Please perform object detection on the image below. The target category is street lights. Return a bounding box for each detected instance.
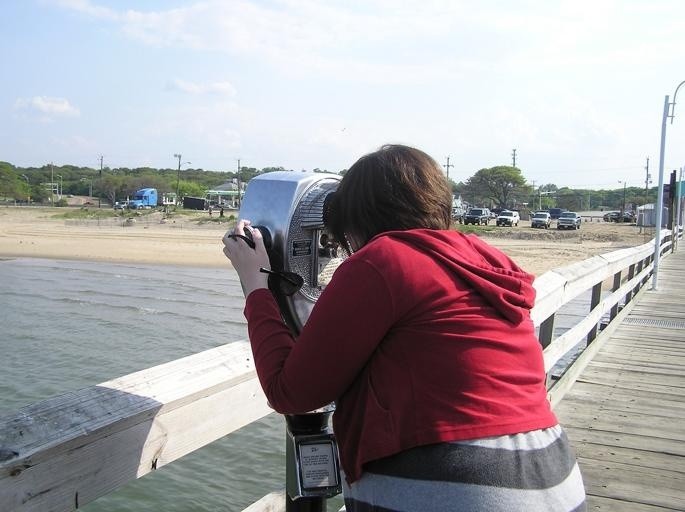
[617,176,631,217]
[93,151,109,189]
[509,146,521,175]
[171,153,193,197]
[641,153,654,210]
[442,156,456,182]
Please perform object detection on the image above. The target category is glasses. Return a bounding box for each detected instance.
[228,225,305,297]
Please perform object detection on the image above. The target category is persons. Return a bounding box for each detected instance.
[221,144,587,512]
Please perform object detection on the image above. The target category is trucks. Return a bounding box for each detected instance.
[130,187,158,210]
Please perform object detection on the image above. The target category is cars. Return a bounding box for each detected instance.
[451,207,583,231]
[113,201,129,210]
[603,211,636,224]
